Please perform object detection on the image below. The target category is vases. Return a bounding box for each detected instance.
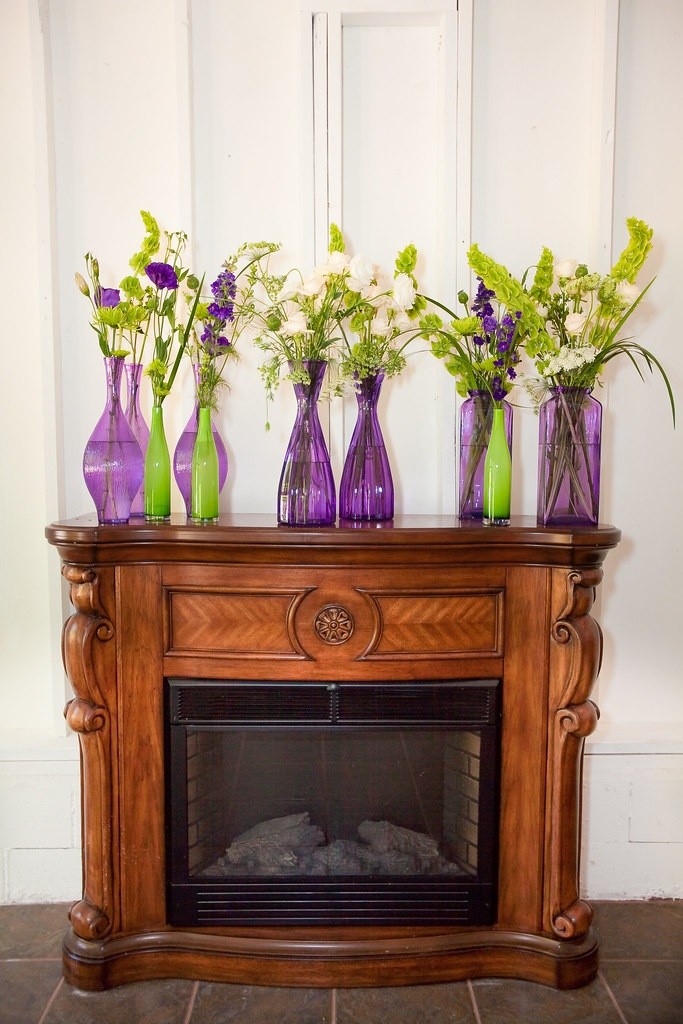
[484,405,513,520]
[455,387,513,520]
[173,401,227,516]
[119,362,148,518]
[339,365,396,523]
[532,385,602,528]
[277,358,337,527]
[146,404,170,516]
[191,406,219,522]
[81,352,143,526]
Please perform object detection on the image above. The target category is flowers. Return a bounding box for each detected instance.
[74,208,676,425]
